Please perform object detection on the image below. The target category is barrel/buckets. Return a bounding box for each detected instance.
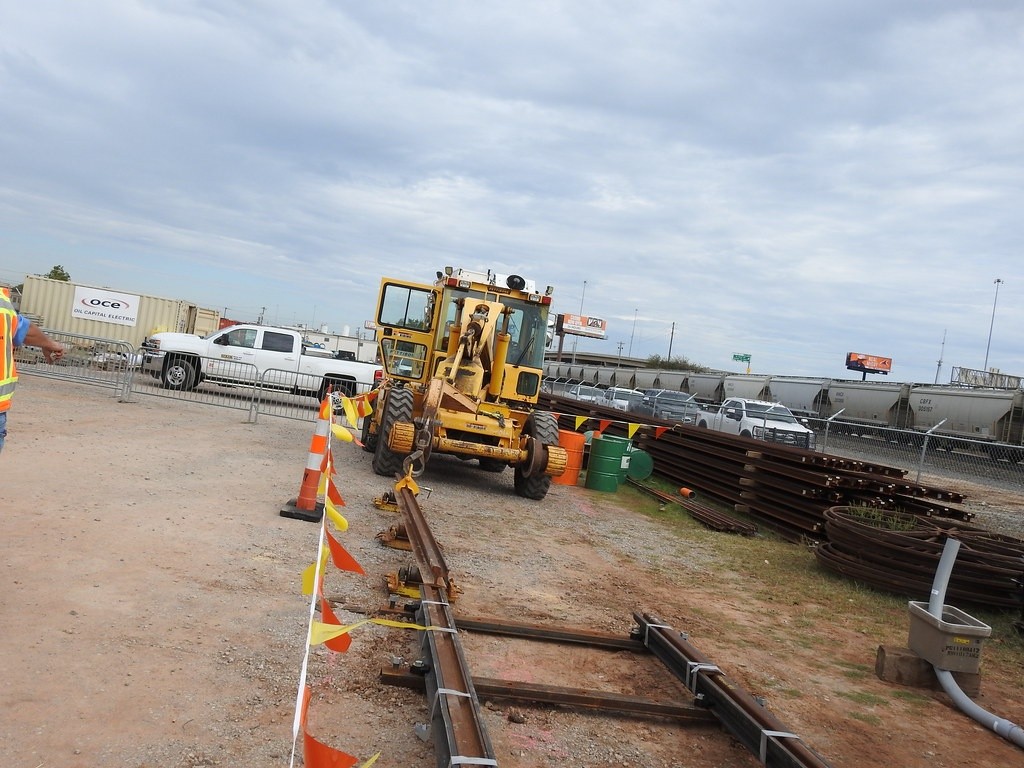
[551,429,586,486]
[603,434,633,485]
[628,447,654,481]
[585,437,625,492]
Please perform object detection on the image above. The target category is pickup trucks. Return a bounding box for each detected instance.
[696,398,816,452]
[594,387,644,417]
[142,325,382,415]
[628,390,695,425]
[562,385,604,404]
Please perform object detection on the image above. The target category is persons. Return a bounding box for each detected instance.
[0,285,63,454]
[850,355,867,368]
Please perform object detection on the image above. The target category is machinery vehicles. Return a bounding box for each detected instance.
[362,266,566,502]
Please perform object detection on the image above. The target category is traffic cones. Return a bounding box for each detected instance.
[279,382,341,523]
